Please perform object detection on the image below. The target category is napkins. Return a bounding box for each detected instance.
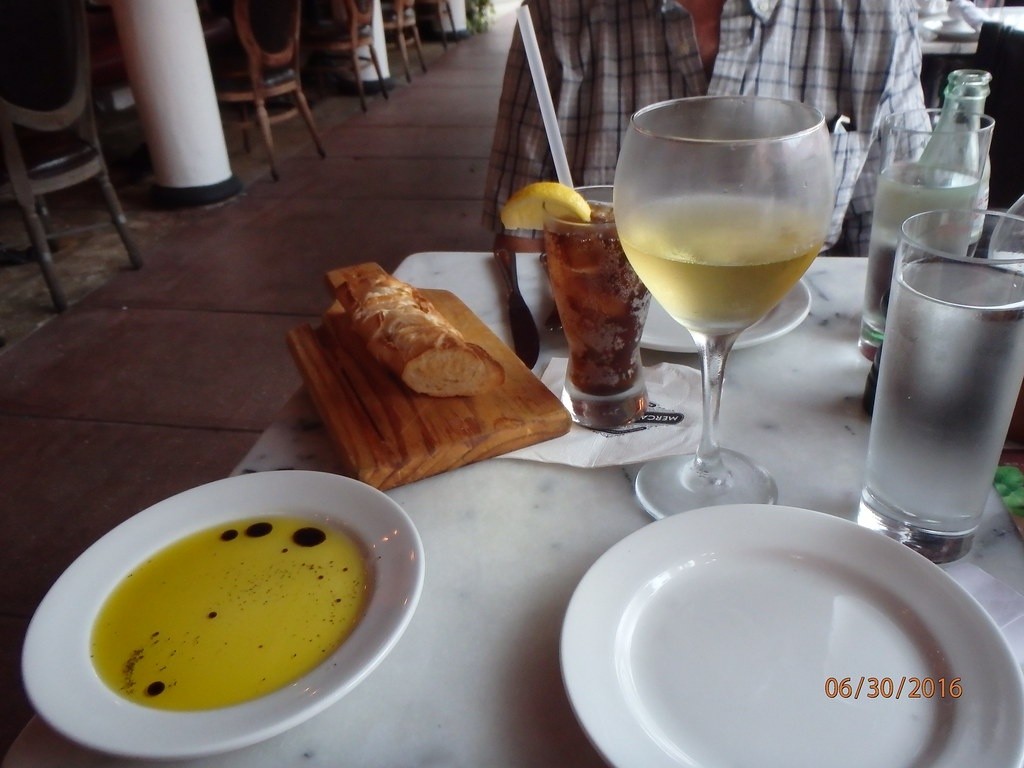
[494,357,725,469]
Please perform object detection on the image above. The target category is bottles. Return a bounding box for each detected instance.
[912,71,997,257]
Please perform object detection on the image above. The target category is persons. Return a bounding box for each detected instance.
[478,0,932,261]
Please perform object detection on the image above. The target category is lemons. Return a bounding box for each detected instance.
[502,182,591,231]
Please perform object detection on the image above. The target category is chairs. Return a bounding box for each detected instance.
[0,0,457,313]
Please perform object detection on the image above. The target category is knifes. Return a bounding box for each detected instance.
[495,250,540,371]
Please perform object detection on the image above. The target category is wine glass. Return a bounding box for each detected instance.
[611,96,835,522]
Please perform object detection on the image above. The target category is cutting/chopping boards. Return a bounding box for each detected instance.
[288,262,571,494]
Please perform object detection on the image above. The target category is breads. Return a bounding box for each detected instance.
[336,273,504,396]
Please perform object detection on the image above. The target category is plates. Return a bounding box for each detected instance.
[21,470,426,761]
[635,277,814,354]
[558,504,1024,768]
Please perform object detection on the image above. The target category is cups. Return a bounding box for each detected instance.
[858,108,995,357]
[856,207,1024,563]
[542,184,648,429]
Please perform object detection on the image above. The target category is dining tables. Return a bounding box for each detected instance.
[0,252,1024,768]
